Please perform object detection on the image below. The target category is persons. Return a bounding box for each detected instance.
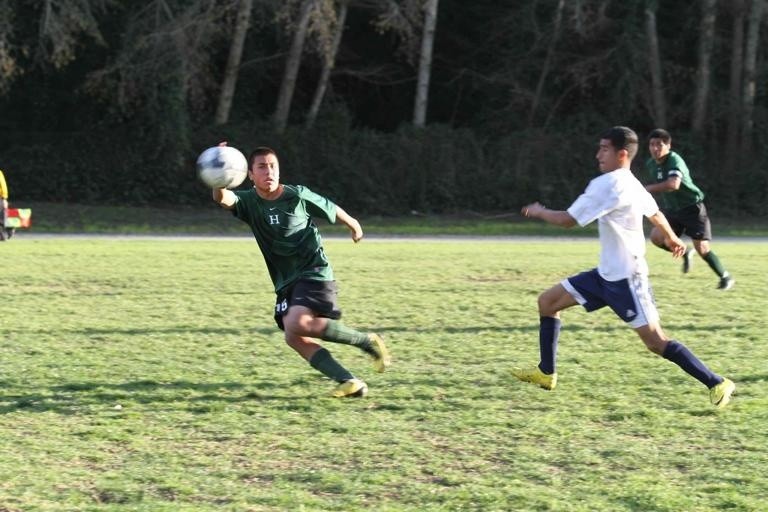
[0,170,15,240]
[509,126,736,412]
[212,141,388,398]
[644,128,735,291]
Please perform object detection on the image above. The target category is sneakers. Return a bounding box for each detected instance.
[716,275,735,289]
[709,377,735,408]
[510,366,557,390]
[683,248,696,273]
[327,378,368,398]
[365,333,388,373]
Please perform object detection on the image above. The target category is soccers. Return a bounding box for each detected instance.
[199,145,248,188]
